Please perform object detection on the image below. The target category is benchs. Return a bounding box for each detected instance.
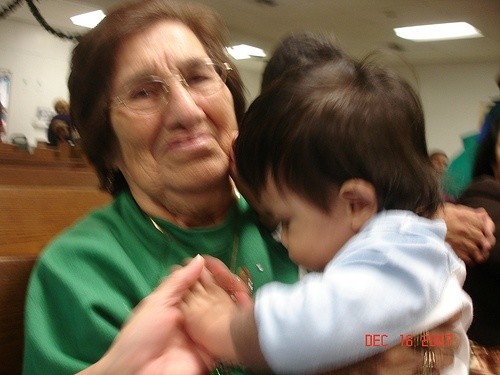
[0,141,116,375]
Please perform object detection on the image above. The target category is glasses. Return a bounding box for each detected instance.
[107,62,231,115]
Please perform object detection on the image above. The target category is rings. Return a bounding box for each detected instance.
[422,350,435,370]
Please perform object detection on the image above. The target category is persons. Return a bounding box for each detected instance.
[19,0,496,375]
[70,253,251,375]
[427,86,499,352]
[166,43,478,375]
[48,100,82,147]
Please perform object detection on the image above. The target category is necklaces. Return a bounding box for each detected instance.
[146,193,242,274]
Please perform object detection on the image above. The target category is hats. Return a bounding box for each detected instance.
[427,141,448,158]
[53,120,67,130]
